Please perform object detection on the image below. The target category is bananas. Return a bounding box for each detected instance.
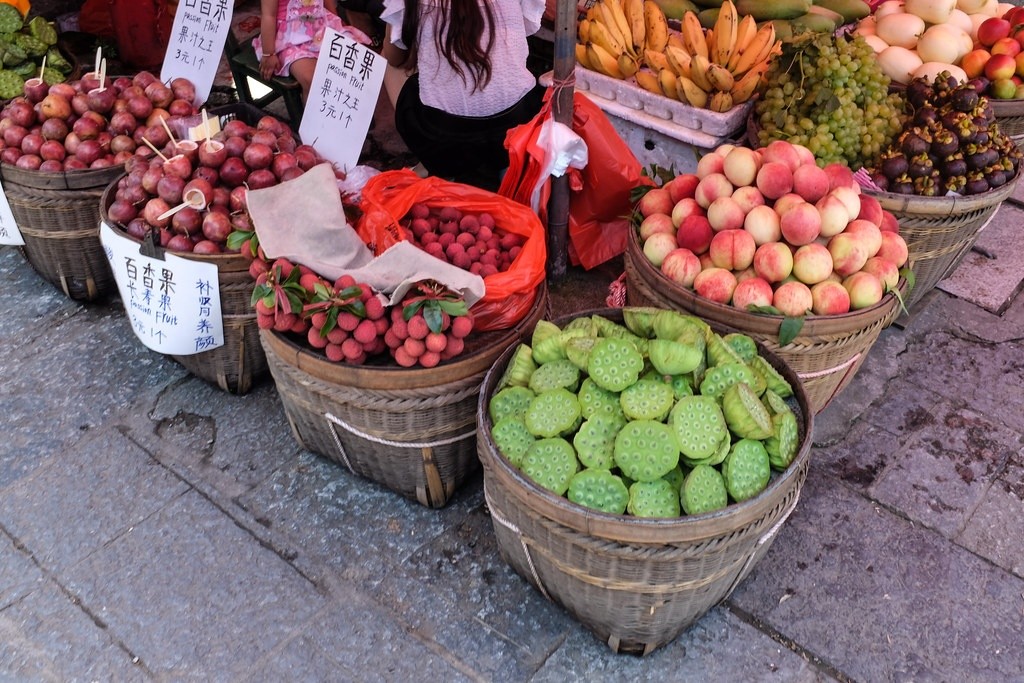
[574,0,785,108]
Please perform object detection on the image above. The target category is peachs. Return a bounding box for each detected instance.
[640,140,907,315]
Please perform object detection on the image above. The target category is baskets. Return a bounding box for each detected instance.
[98,130,339,396]
[834,0,1024,151]
[253,206,551,510]
[14,26,127,84]
[624,178,908,418]
[0,75,163,300]
[748,89,1024,313]
[476,305,816,657]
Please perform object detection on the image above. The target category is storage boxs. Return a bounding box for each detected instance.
[539,69,746,185]
[574,59,753,136]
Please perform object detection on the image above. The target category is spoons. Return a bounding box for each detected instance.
[201,107,216,154]
[156,192,203,222]
[158,114,184,149]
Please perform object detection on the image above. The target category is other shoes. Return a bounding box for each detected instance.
[402,166,456,182]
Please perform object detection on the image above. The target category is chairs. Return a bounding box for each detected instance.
[221,0,303,131]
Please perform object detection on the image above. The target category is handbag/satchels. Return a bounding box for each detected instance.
[358,166,546,329]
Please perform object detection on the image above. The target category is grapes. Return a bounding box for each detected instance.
[752,30,913,169]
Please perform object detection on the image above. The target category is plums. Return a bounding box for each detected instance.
[961,6,1023,100]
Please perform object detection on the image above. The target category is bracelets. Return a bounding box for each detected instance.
[261,53,275,56]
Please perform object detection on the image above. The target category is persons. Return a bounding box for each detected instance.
[379,0,546,179]
[252,0,374,155]
